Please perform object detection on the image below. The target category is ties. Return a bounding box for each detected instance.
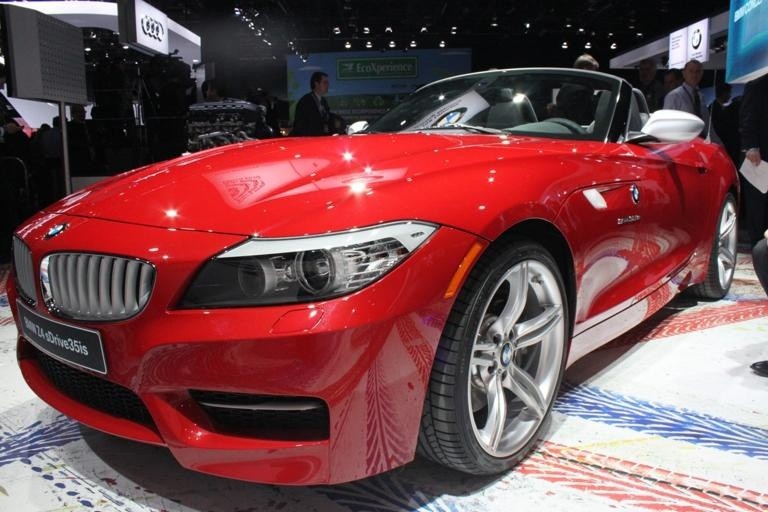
[691,89,701,117]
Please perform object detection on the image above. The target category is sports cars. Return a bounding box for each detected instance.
[5,68,745,489]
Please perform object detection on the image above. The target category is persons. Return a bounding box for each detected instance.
[0,62,97,263]
[206,78,232,99]
[292,71,333,136]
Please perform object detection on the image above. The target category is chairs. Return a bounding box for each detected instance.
[486,89,538,133]
[591,86,650,142]
